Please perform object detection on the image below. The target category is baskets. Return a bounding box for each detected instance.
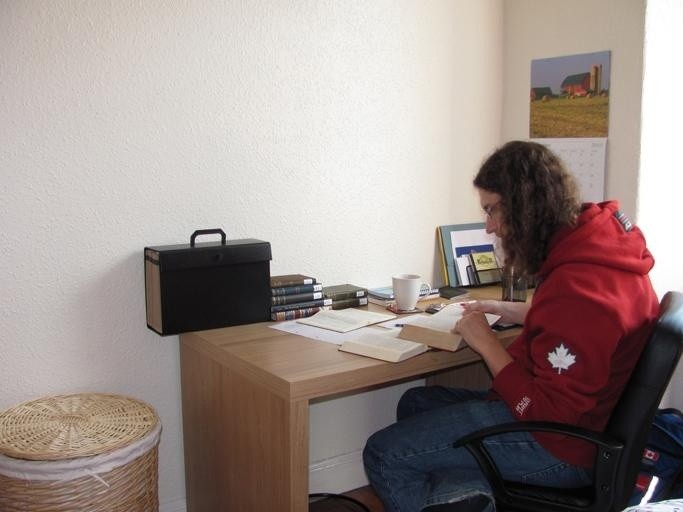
[0,393,162,512]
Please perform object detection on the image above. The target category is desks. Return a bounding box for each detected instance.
[177,288,548,512]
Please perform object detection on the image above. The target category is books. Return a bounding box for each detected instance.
[336,320,432,361]
[439,251,502,299]
[399,303,503,354]
[271,274,370,321]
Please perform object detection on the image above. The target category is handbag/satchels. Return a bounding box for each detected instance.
[627,407,683,505]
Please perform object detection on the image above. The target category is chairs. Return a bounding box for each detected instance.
[452,291,682,511]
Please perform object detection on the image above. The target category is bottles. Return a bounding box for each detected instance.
[500,256,528,303]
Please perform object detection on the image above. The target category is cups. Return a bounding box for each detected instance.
[391,274,432,312]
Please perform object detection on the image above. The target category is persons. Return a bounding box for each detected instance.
[363,141,660,511]
